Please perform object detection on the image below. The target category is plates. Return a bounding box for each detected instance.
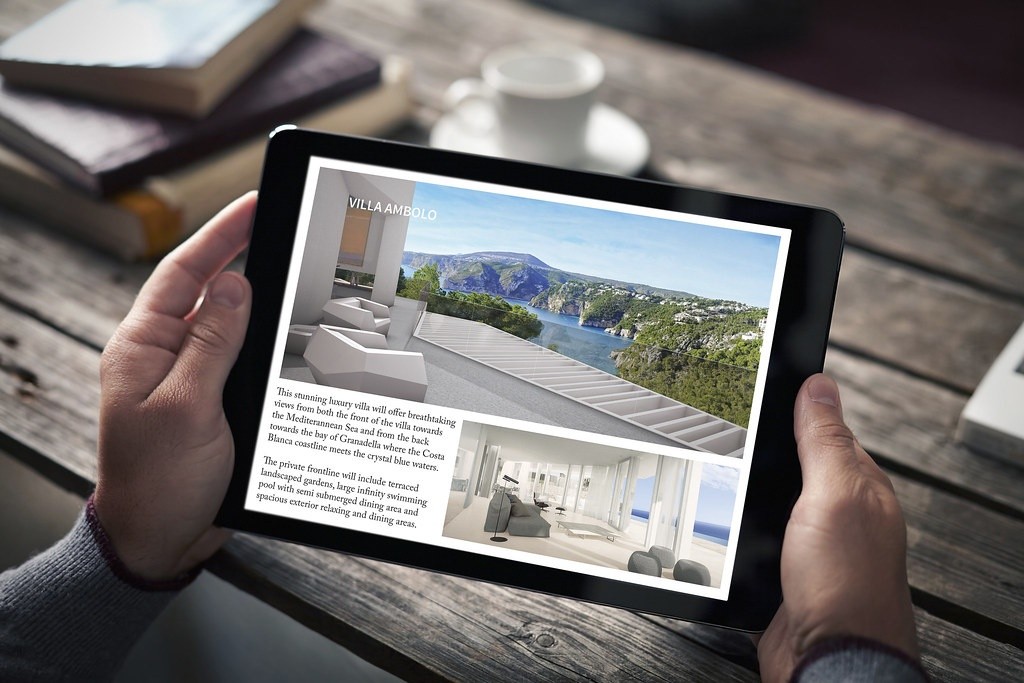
[425,96,656,182]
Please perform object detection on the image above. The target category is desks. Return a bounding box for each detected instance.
[2,1,1024,683]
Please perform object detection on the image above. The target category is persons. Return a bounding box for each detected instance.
[0,192,934,682]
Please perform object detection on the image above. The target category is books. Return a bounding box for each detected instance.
[1,2,411,263]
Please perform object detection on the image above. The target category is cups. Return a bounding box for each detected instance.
[445,39,606,169]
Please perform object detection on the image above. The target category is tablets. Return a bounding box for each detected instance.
[210,125,847,631]
